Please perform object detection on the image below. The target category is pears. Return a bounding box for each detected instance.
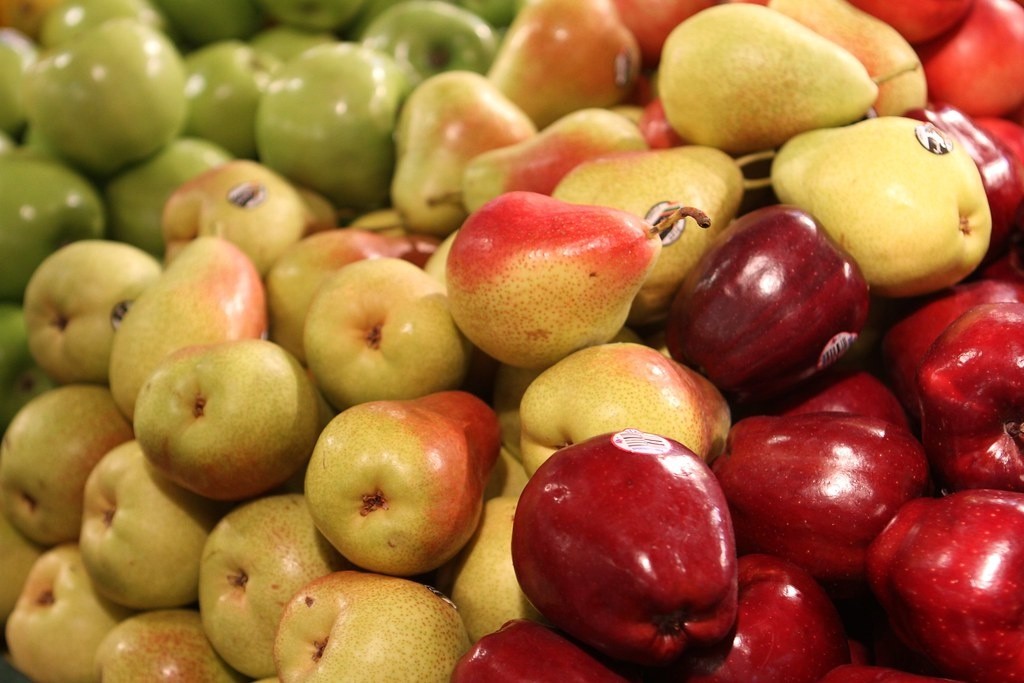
[0,0,992,683]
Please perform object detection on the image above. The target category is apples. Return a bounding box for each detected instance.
[451,1,1024,683]
